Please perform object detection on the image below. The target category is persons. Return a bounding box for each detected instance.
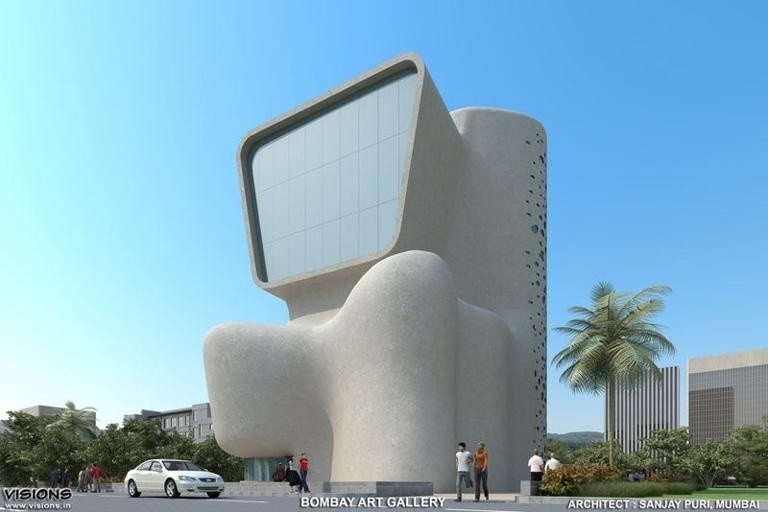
[296,451,312,493]
[288,454,300,494]
[544,452,561,473]
[527,448,544,480]
[472,439,490,503]
[452,441,474,502]
[48,462,101,492]
